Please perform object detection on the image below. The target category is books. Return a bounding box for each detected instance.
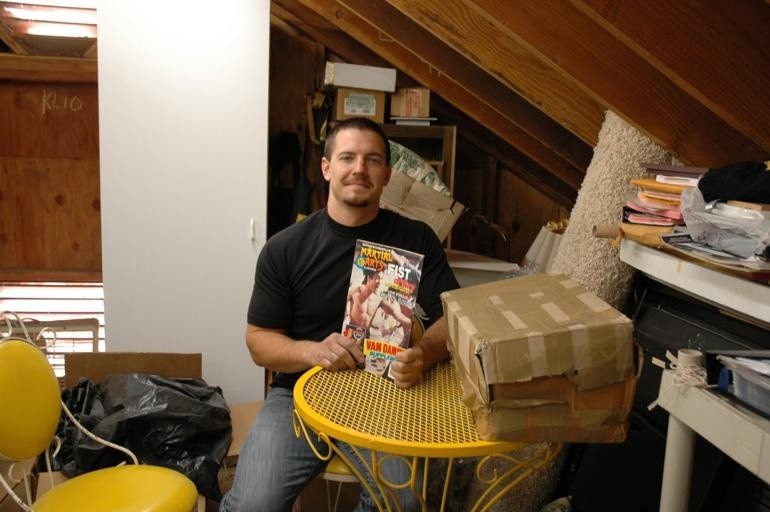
[322,57,432,126]
[436,272,636,445]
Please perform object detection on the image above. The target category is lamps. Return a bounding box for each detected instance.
[0,313,202,511]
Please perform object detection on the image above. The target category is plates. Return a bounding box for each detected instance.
[620,238,770,511]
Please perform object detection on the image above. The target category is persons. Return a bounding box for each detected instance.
[219,116,460,512]
[346,270,412,352]
[381,287,403,328]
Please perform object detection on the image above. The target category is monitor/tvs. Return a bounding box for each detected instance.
[340,238,426,383]
